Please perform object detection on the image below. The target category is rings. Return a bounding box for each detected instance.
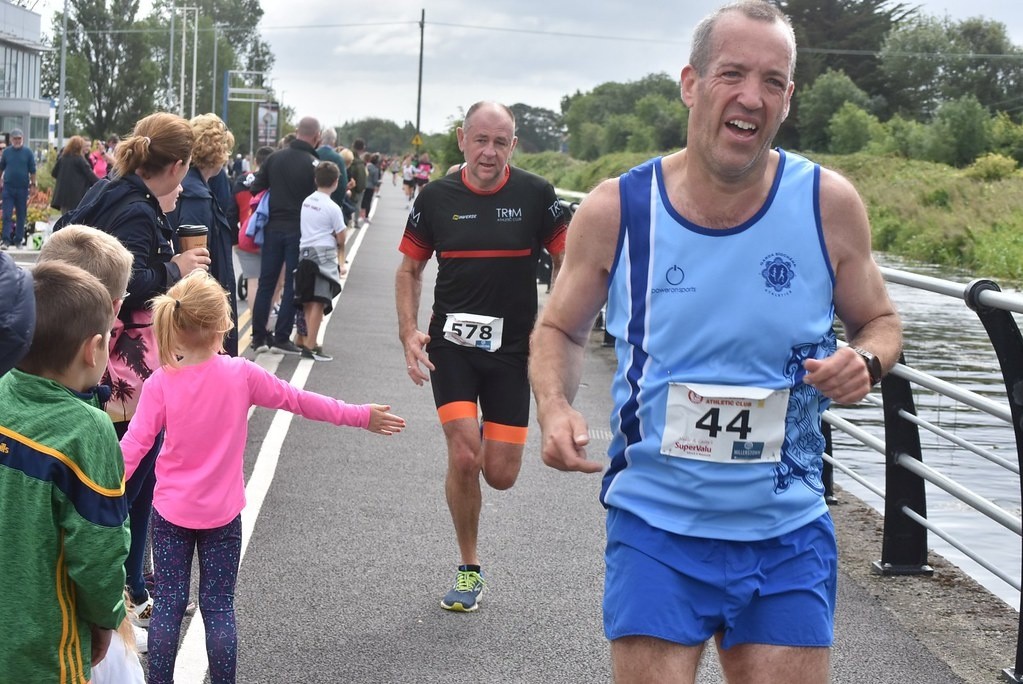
[408,365,412,369]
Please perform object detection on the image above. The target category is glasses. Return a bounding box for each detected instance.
[13,137,22,140]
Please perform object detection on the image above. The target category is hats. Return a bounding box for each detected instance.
[10,128,23,138]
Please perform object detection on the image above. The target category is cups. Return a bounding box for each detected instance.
[175,224,209,254]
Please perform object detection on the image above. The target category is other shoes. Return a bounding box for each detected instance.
[405,205,410,210]
[1,241,10,250]
[13,236,22,250]
[364,217,373,224]
[123,585,154,628]
[354,224,361,229]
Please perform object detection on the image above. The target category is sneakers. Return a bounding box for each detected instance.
[294,345,304,351]
[254,340,269,353]
[304,349,333,361]
[440,571,490,612]
[271,344,304,355]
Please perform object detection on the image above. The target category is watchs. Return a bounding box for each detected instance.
[854,347,882,386]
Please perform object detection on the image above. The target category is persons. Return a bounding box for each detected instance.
[394,100,570,610]
[119,269,406,684]
[233,117,388,361]
[0,112,243,684]
[390,151,434,210]
[529,0,903,684]
